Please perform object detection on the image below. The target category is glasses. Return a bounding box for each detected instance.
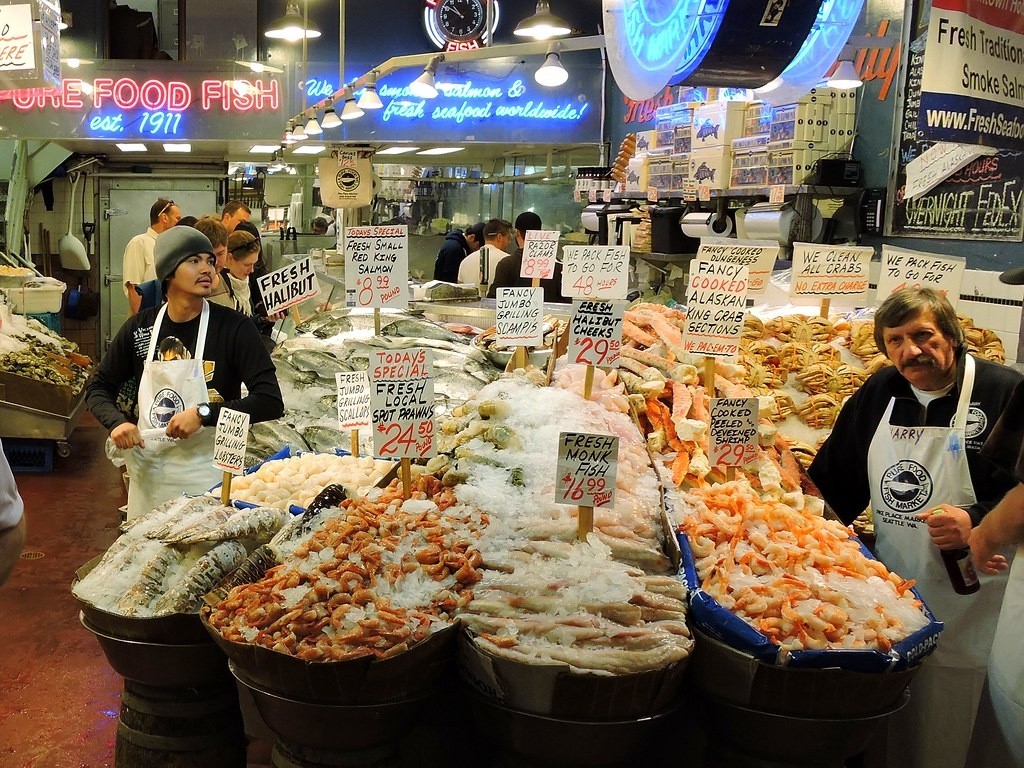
[157,199,174,219]
[230,236,261,256]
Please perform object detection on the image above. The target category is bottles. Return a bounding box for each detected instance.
[665,298,687,313]
[574,167,618,192]
[932,509,981,595]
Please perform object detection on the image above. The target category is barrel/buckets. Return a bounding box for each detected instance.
[268,733,409,768]
[114,679,249,768]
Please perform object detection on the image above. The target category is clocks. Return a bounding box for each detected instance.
[423,0,500,52]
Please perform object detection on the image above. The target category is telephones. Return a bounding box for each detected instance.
[856,184,883,233]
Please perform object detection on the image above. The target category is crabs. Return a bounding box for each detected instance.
[633,304,1008,486]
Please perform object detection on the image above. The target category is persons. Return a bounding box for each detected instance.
[0,438,26,587]
[486,212,572,304]
[808,288,1024,768]
[175,200,289,356]
[312,207,336,235]
[457,218,513,298]
[433,222,486,284]
[85,226,285,522]
[123,199,182,321]
[967,377,1024,768]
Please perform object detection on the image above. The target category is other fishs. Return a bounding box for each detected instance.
[219,295,569,490]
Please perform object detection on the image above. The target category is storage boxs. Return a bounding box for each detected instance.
[0,276,68,335]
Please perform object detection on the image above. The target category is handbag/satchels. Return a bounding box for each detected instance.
[64,274,98,321]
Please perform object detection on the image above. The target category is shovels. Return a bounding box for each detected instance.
[58,171,91,271]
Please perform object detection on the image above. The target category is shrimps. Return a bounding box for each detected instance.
[82,488,923,671]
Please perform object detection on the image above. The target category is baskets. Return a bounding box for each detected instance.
[25,312,61,337]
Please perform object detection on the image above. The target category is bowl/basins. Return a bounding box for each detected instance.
[683,685,911,760]
[463,688,688,768]
[225,659,456,752]
[78,609,228,687]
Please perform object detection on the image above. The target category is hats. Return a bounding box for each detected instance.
[154,223,216,287]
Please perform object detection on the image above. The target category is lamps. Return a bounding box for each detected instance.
[60,55,96,68]
[513,0,571,38]
[281,118,298,144]
[303,105,323,134]
[827,36,900,88]
[408,54,445,98]
[289,112,309,140]
[321,95,343,129]
[267,144,287,171]
[1,437,55,473]
[340,83,365,120]
[626,85,856,193]
[356,70,383,110]
[534,41,569,87]
[264,0,322,42]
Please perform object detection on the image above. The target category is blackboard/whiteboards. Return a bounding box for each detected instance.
[884,2,1021,243]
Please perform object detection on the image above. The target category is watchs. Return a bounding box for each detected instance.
[197,402,212,427]
